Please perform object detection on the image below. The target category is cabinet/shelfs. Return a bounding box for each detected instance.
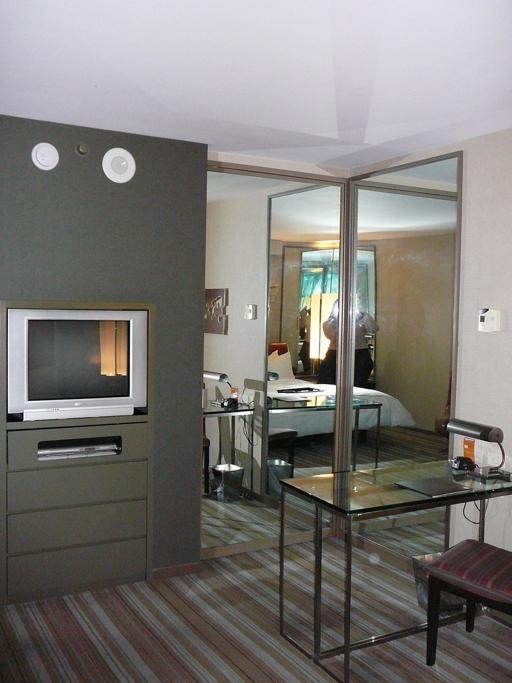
[0,298,156,605]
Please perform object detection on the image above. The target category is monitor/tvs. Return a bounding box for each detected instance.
[8,309,147,422]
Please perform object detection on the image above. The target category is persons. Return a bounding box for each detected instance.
[318,292,374,389]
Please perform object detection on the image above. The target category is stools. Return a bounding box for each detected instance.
[267,424,298,479]
[424,538,512,667]
[202,436,211,494]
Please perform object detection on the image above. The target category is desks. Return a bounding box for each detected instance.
[201,397,256,501]
[277,456,512,683]
[268,394,384,472]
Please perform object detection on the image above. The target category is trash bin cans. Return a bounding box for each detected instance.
[266,459,292,495]
[213,463,244,501]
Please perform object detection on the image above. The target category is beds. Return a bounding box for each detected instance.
[267,343,416,452]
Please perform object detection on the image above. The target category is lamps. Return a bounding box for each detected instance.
[443,419,506,480]
[201,369,239,409]
[266,369,280,382]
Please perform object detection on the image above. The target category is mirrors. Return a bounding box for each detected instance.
[260,183,344,529]
[200,158,349,562]
[347,150,464,537]
[344,177,457,533]
